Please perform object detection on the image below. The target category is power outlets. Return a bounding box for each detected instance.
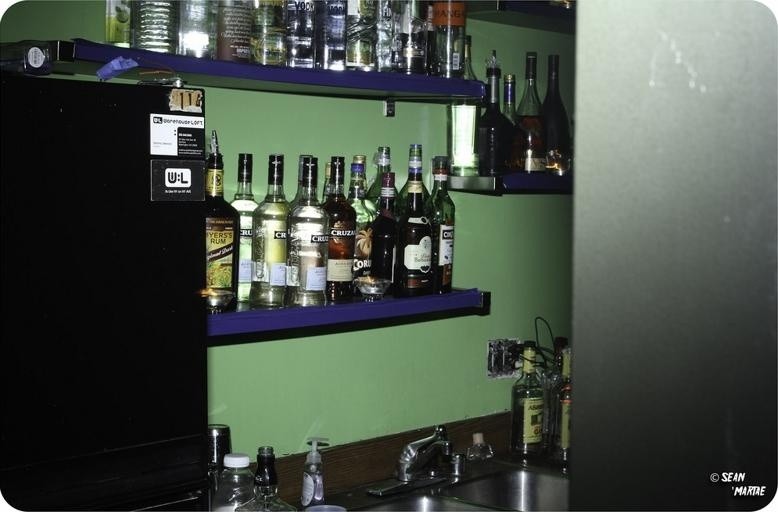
[485,338,522,381]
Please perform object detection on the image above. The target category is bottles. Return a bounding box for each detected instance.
[204,143,458,311]
[447,34,570,177]
[232,445,299,512]
[466,433,494,459]
[511,333,571,471]
[131,0,471,80]
[212,451,255,512]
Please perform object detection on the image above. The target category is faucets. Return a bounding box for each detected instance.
[391,431,452,483]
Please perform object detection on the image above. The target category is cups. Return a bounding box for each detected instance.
[306,504,346,511]
[207,422,232,490]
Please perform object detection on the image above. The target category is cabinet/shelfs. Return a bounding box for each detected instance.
[445,1,577,197]
[0,34,486,338]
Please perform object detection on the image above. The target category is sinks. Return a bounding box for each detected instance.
[442,469,569,512]
[366,494,493,512]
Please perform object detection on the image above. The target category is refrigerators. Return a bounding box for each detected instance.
[1,75,204,508]
